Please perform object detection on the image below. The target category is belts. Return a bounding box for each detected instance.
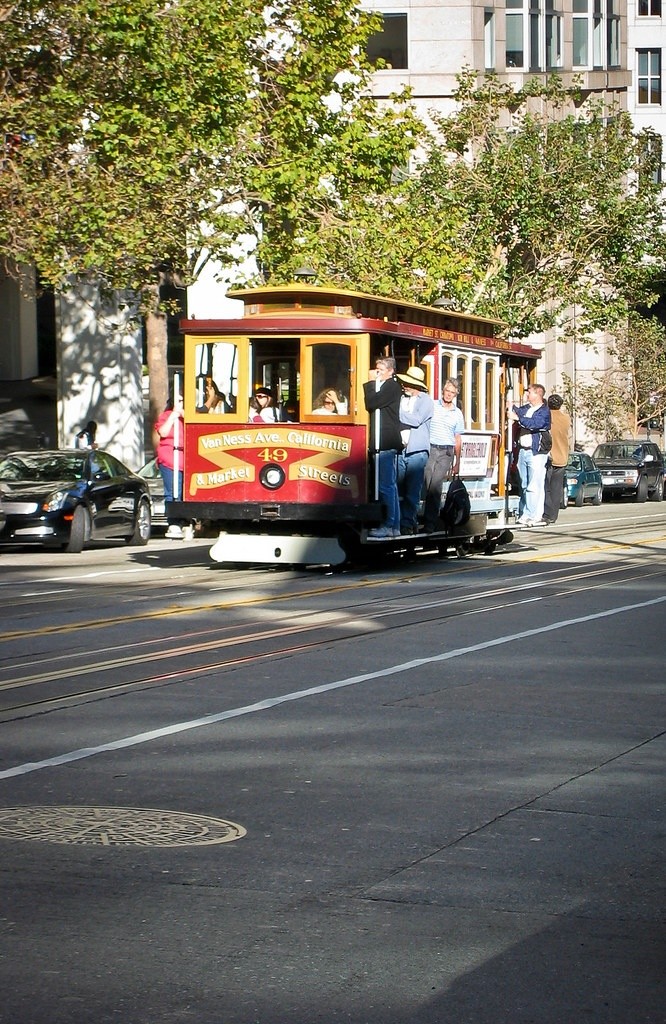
[521,446,532,450]
[431,445,454,449]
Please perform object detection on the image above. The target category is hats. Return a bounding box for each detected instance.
[396,366,428,388]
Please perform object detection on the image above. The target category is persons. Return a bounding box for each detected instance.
[363,356,404,536]
[155,390,193,542]
[506,383,551,525]
[249,388,279,423]
[396,367,434,535]
[312,388,348,415]
[542,394,571,523]
[418,378,465,534]
[197,375,230,413]
[75,420,98,467]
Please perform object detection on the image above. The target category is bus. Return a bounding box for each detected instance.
[165,285,542,574]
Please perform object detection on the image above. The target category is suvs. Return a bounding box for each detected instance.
[592,440,664,503]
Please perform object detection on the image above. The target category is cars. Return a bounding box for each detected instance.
[137,456,207,538]
[564,452,603,506]
[0,449,152,553]
[490,450,568,510]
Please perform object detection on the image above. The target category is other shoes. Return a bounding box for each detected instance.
[418,528,435,534]
[516,514,531,523]
[165,525,190,538]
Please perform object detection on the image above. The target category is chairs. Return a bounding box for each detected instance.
[633,447,643,458]
[572,461,580,468]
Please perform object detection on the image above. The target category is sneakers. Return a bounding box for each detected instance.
[401,528,413,534]
[370,527,393,536]
[393,529,401,536]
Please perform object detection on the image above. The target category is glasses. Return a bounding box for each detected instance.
[256,395,268,398]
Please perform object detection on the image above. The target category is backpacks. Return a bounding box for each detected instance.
[444,473,470,527]
